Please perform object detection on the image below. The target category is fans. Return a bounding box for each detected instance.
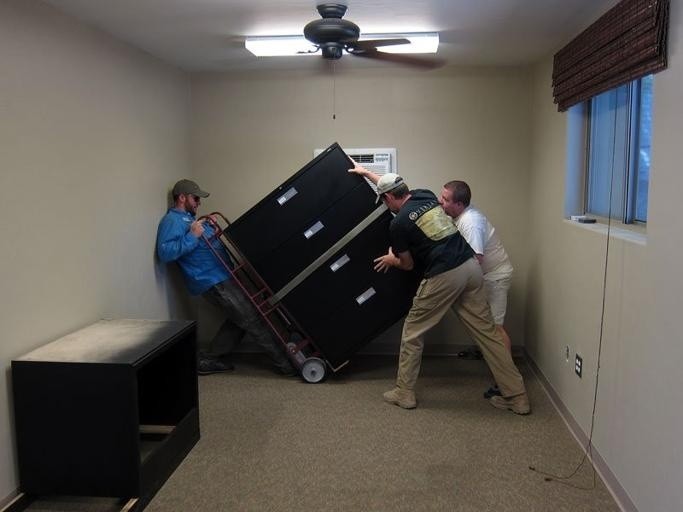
[296,3,447,70]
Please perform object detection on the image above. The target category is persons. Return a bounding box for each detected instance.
[347,154,530,414]
[437,180,514,399]
[155,178,302,378]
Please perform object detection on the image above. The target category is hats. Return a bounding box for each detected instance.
[172,180,210,199]
[374,173,404,205]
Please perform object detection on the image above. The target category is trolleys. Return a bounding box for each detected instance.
[194,211,351,386]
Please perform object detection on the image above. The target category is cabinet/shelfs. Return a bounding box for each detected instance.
[10,316,200,498]
[220,141,424,381]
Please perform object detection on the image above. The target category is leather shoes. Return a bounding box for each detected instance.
[457,350,482,360]
[484,388,501,397]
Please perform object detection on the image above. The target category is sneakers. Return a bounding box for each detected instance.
[490,393,531,415]
[280,367,298,377]
[198,351,234,375]
[383,387,417,409]
